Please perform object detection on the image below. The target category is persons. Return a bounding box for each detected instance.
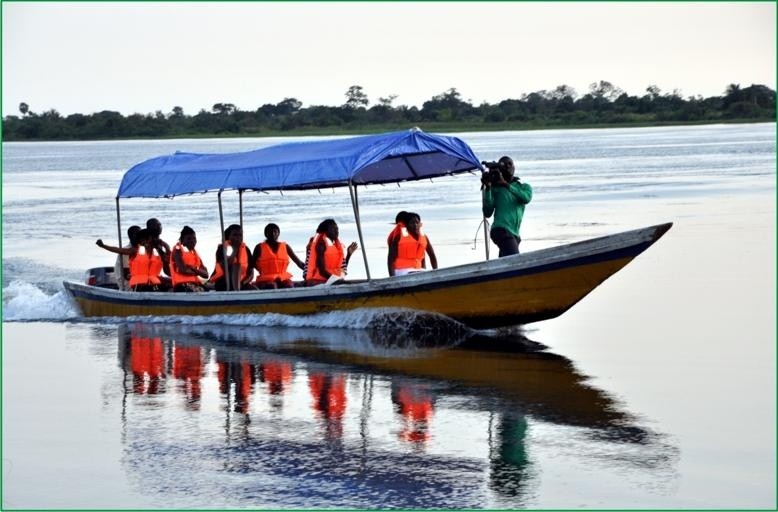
[257,357,295,409]
[388,211,426,270]
[253,223,305,289]
[390,377,436,443]
[387,212,437,277]
[303,219,358,287]
[307,366,348,439]
[118,322,256,425]
[170,226,209,293]
[488,408,531,498]
[96,218,170,292]
[208,224,254,291]
[481,156,532,258]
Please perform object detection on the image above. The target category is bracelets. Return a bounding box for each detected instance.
[196,266,200,272]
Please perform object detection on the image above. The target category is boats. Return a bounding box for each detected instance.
[129,323,653,445]
[58,222,676,330]
